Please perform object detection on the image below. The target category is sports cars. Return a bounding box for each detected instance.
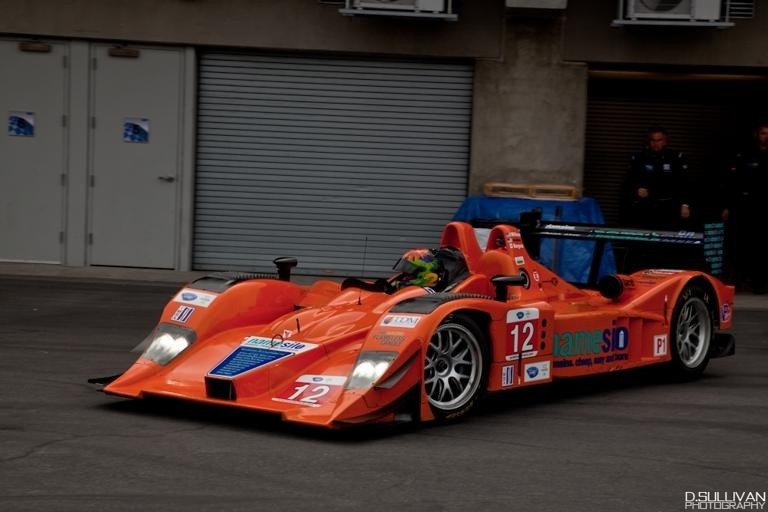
[85,206,739,436]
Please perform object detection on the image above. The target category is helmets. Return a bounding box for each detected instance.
[392,248,442,290]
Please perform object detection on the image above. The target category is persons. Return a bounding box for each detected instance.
[624,123,768,294]
